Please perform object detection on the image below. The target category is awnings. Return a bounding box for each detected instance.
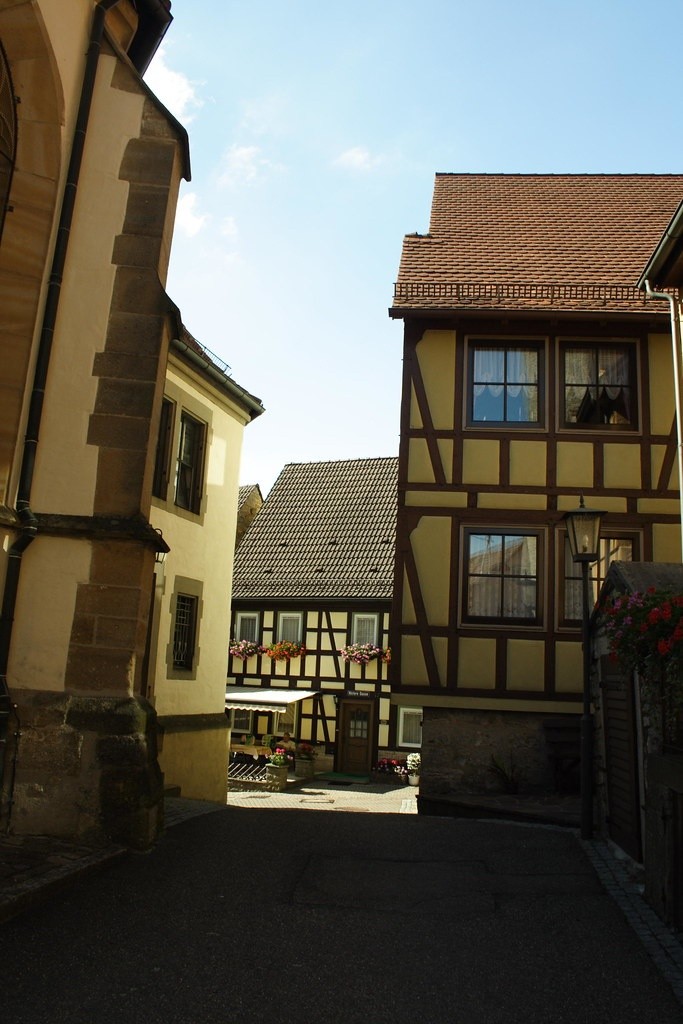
[225,685,321,713]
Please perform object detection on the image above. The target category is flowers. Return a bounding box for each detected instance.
[594,585,683,676]
[381,646,393,664]
[269,748,294,769]
[229,638,265,661]
[341,642,380,664]
[378,753,421,774]
[266,639,305,662]
[298,743,316,760]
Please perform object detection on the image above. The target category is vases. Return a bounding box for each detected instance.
[408,774,419,785]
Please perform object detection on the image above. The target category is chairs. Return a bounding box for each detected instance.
[241,735,255,744]
[261,734,274,746]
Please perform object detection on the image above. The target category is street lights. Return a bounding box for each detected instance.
[559,494,609,840]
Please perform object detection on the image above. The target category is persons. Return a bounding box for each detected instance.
[276,732,296,753]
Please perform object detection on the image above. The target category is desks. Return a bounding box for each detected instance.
[230,744,273,766]
